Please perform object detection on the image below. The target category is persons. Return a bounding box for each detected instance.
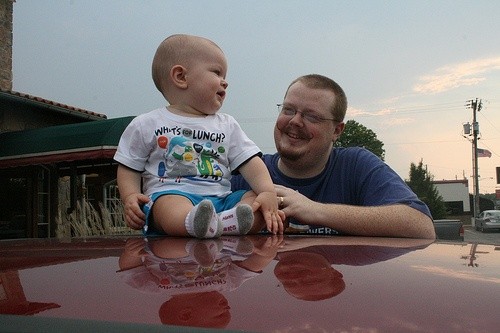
[116,236,286,328]
[272,237,438,301]
[230,74,436,239]
[113,34,285,238]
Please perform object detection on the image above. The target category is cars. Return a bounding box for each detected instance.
[475,210,500,233]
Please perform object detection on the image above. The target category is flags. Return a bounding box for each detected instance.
[477,148,492,158]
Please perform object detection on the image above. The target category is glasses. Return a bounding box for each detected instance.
[277,102,341,125]
